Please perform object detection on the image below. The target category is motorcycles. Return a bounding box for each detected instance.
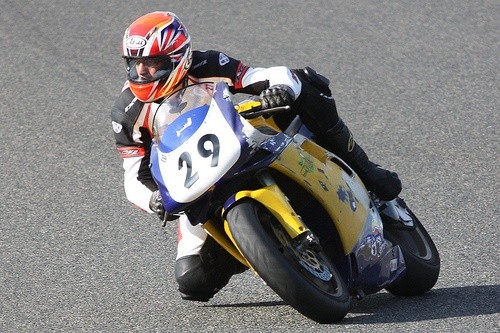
[134,81,442,324]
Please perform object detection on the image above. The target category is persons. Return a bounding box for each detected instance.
[110,11,403,301]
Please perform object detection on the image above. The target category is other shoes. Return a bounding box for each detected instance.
[361,160,401,199]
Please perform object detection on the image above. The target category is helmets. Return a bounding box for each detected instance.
[123,10,193,103]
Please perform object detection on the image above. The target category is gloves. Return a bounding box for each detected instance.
[259,84,296,119]
[149,188,181,221]
